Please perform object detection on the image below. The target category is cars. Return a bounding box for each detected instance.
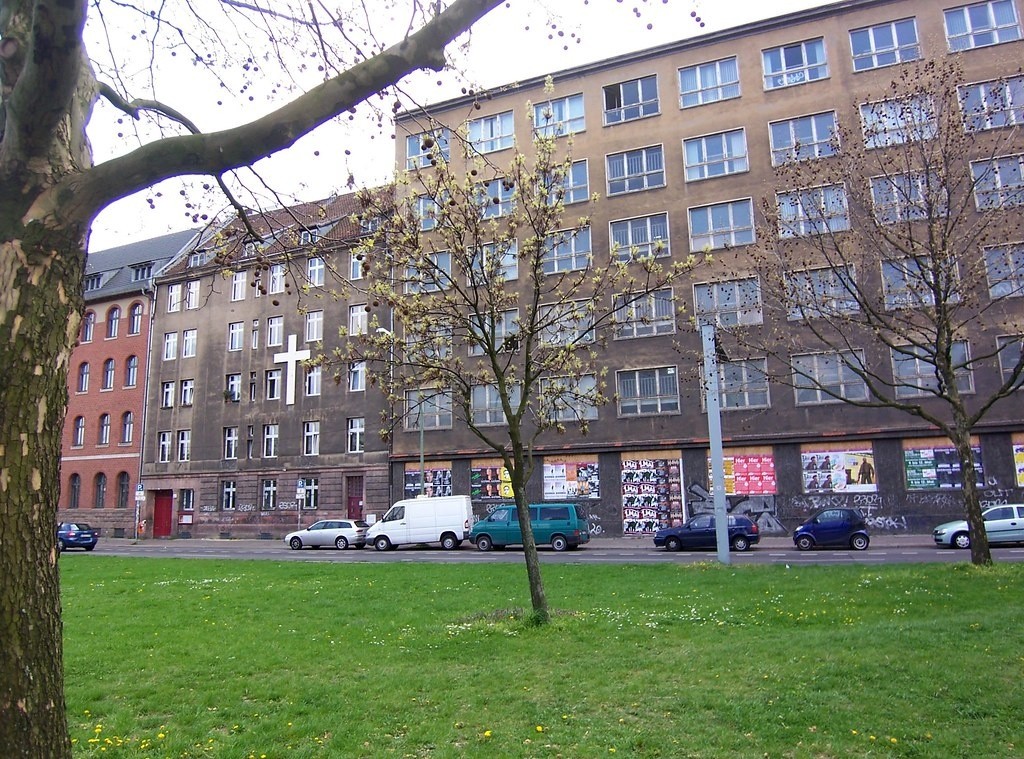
[793,507,871,552]
[932,504,1023,549]
[285,519,371,550]
[653,513,761,552]
[57,521,99,552]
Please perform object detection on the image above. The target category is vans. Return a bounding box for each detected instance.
[468,504,591,551]
[365,495,473,551]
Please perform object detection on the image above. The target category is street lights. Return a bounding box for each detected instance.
[397,397,426,495]
